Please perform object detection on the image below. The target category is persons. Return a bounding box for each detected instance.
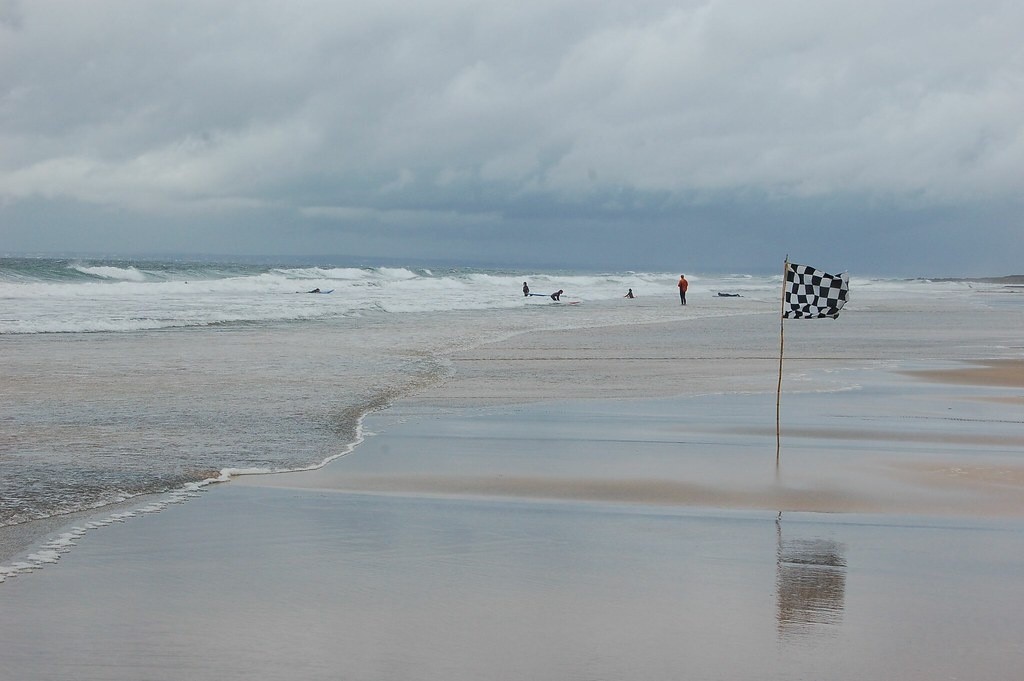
[718,291,742,299]
[523,282,530,297]
[624,288,635,299]
[677,274,689,306]
[551,290,563,302]
[296,288,320,294]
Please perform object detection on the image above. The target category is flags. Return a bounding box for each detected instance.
[782,262,849,321]
